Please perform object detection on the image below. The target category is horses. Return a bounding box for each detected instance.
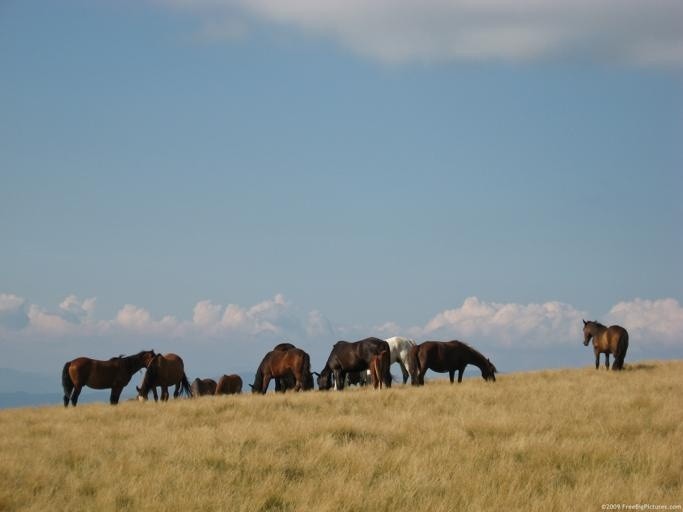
[62,348,157,408]
[583,319,629,371]
[314,336,419,390]
[189,377,217,397]
[411,340,497,387]
[214,374,243,395]
[248,343,315,395]
[135,353,194,402]
